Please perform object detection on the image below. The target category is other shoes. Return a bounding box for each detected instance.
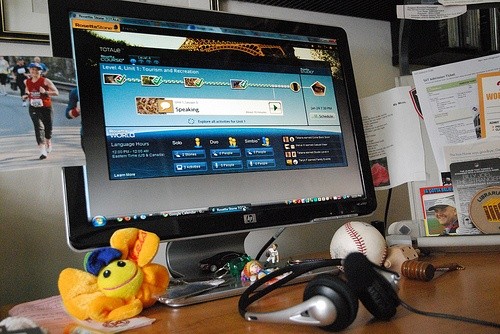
[39,150,47,159]
[23,102,27,107]
[46,145,51,152]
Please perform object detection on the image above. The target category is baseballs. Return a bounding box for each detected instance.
[330,222,385,274]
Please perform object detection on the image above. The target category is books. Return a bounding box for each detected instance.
[436,6,499,53]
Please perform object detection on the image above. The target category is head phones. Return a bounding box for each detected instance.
[238,251,401,334]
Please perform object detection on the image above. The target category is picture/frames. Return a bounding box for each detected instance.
[0,0,220,46]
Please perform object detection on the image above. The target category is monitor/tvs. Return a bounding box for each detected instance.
[47,0,378,308]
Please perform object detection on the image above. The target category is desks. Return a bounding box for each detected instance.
[0,248,499,334]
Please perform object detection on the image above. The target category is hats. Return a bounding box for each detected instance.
[428,198,455,211]
[18,57,25,60]
[30,63,43,70]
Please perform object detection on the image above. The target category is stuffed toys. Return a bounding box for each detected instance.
[239,259,280,282]
[57,228,170,323]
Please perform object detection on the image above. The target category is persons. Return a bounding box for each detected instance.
[1,56,10,96]
[32,56,50,77]
[264,243,279,266]
[65,87,85,154]
[22,63,60,158]
[13,58,29,107]
[428,198,458,233]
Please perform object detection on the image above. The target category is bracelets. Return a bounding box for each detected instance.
[72,108,80,118]
